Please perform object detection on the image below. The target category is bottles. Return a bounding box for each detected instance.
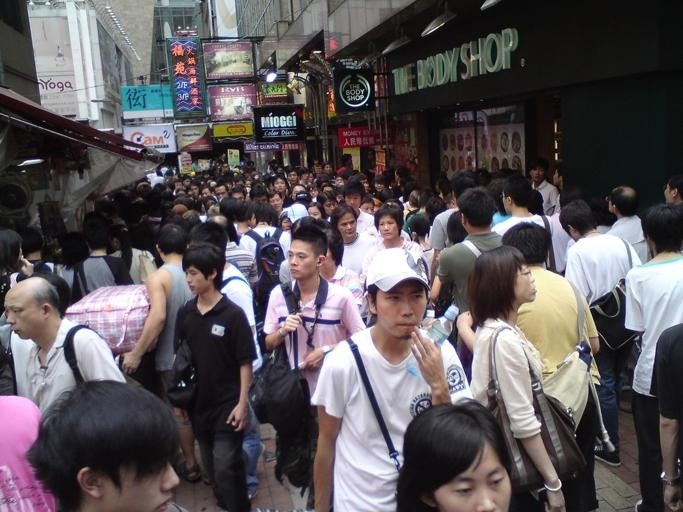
[405,303,460,380]
[421,308,436,326]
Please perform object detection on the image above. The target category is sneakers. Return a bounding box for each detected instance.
[595,444,622,466]
[635,500,644,512]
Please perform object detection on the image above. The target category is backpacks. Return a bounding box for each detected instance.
[245,227,285,316]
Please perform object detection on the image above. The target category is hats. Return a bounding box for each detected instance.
[360,248,431,300]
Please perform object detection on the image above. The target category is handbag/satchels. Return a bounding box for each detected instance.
[274,430,318,497]
[588,286,639,351]
[542,341,592,430]
[166,340,197,410]
[64,285,160,355]
[488,379,588,493]
[249,360,310,434]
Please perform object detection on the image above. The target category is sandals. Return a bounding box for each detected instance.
[175,452,201,483]
